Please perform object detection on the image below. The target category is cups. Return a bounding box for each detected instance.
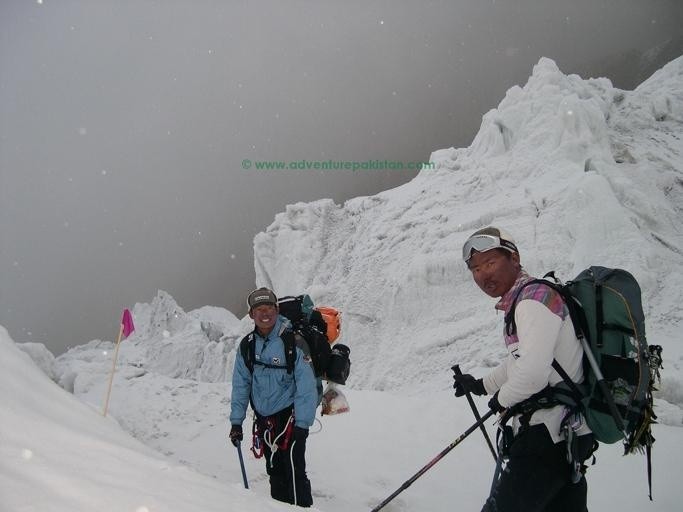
[463,235,500,263]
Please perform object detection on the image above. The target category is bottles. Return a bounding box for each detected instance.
[462,227,518,263]
[247,286,279,312]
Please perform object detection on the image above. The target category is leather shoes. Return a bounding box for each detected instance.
[291,426,311,443]
[488,388,509,416]
[452,372,489,398]
[230,422,244,447]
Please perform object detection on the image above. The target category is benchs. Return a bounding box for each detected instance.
[121,307,135,338]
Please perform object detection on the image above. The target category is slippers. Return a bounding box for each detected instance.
[240,295,332,408]
[505,265,655,444]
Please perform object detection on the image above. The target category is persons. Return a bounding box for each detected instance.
[227,286,314,509]
[450,225,597,511]
